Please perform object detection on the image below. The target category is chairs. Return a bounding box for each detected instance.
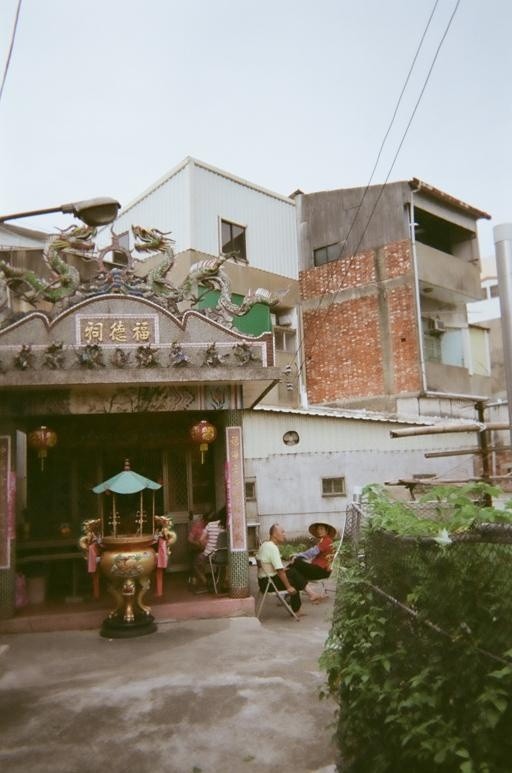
[201,532,229,597]
[252,553,299,623]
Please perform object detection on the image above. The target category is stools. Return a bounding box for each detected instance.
[301,578,329,604]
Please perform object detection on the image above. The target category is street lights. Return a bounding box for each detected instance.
[1,197,122,226]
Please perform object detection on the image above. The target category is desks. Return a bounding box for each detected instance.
[16,536,80,602]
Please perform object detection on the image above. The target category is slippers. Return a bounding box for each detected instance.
[312,595,329,605]
[293,609,308,616]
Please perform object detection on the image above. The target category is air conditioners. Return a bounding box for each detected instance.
[427,317,444,333]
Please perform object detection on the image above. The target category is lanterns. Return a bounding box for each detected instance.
[27,425,58,472]
[190,420,218,465]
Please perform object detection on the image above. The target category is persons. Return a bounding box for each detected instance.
[189,504,225,595]
[187,511,216,563]
[256,524,329,617]
[287,523,337,580]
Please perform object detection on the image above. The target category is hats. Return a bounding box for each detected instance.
[309,521,338,539]
